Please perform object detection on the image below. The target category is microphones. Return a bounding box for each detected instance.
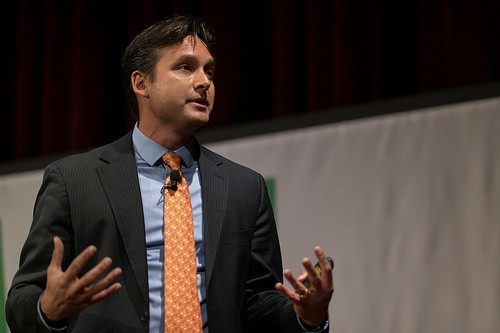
[170,169,180,189]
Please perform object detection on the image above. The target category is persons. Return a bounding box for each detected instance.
[5,12,334,333]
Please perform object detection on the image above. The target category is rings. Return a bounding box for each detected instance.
[300,291,310,299]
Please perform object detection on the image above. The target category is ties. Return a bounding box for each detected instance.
[163,150,203,333]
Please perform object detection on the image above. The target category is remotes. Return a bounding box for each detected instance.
[302,256,333,289]
